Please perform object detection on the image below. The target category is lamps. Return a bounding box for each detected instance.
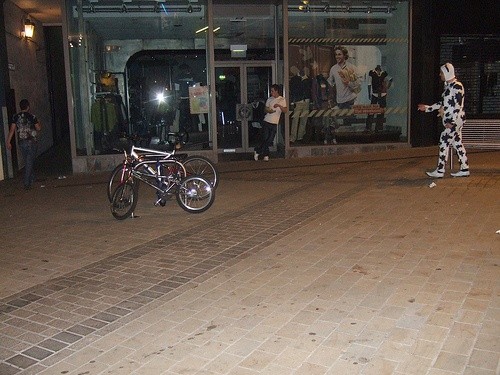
[19,18,36,42]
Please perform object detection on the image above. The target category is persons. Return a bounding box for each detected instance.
[327,46,362,125]
[254,84,288,161]
[7,99,41,190]
[417,62,470,178]
[364,65,393,133]
[311,73,337,145]
[289,65,311,145]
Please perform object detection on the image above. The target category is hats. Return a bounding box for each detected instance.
[440,62,455,81]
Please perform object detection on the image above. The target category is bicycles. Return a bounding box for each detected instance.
[101,131,222,219]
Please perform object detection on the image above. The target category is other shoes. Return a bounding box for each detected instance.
[450,170,470,177]
[263,156,269,161]
[254,149,261,160]
[425,169,444,178]
